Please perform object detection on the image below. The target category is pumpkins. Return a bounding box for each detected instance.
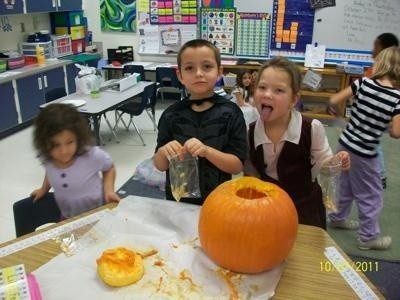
[199,176,299,275]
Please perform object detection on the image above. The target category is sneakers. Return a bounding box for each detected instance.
[359,237,391,250]
[327,219,359,229]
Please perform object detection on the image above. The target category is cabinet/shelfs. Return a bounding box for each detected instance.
[0,54,99,140]
[0,0,24,15]
[25,0,82,14]
[220,64,364,120]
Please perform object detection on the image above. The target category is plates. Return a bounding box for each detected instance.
[60,100,86,107]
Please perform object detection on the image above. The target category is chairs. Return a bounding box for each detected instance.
[109,82,159,146]
[156,67,184,102]
[123,64,146,81]
[12,192,61,238]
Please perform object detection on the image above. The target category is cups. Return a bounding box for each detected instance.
[36,46,45,65]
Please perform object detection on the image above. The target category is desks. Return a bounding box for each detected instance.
[40,78,160,145]
[101,60,179,81]
[0,200,387,300]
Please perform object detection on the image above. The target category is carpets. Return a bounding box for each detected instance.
[324,126,400,262]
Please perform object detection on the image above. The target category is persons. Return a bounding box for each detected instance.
[364,33,398,188]
[327,47,400,250]
[230,70,252,107]
[154,40,246,206]
[243,58,351,231]
[31,103,120,221]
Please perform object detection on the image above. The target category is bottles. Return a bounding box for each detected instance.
[342,62,348,73]
[336,65,345,74]
